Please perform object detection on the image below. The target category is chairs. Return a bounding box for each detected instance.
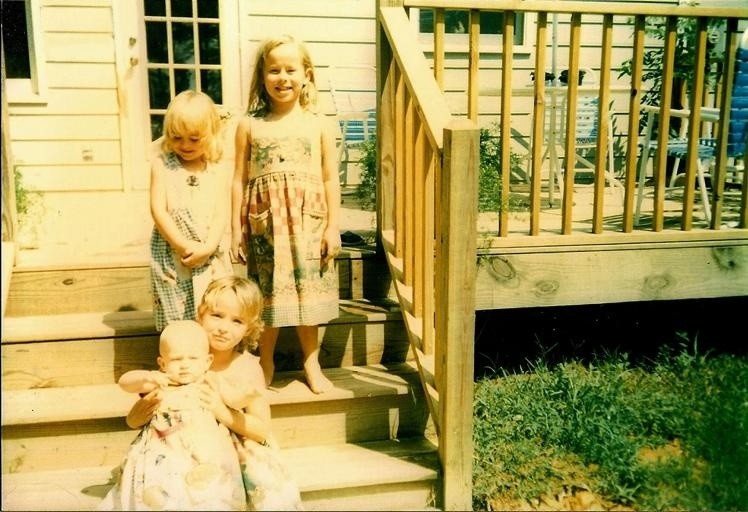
[331,64,383,214]
[523,64,627,208]
[637,42,746,228]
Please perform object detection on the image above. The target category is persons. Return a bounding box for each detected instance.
[228,33,341,396]
[147,90,236,333]
[97,319,267,512]
[94,275,302,511]
